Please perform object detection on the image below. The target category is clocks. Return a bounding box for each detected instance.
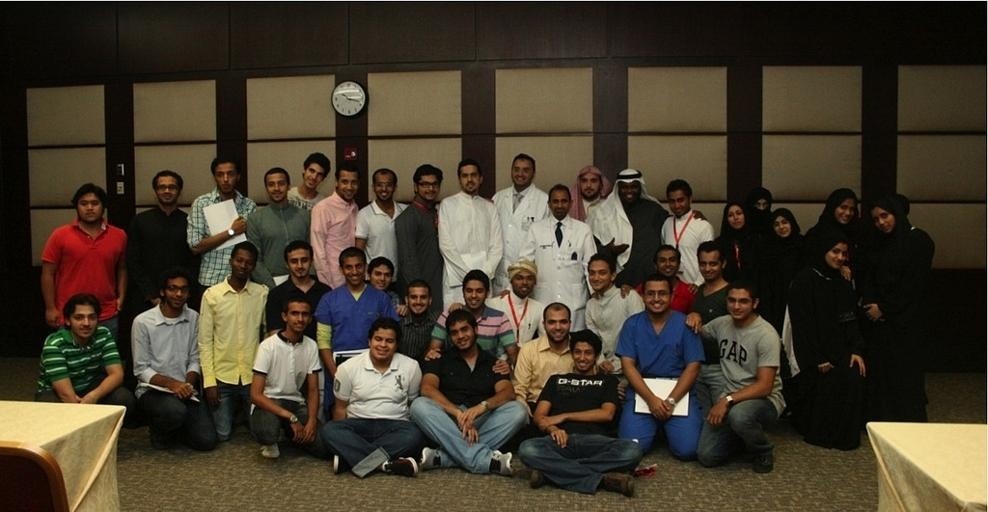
[330,80,369,119]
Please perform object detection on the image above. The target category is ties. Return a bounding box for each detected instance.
[555,222,563,246]
[513,193,522,211]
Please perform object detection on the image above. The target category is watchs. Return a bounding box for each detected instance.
[665,397,676,407]
[288,414,297,425]
[227,227,235,238]
[480,400,489,413]
[725,395,734,407]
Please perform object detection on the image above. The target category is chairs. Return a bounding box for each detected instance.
[0,440,70,512]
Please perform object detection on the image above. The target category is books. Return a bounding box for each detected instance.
[203,198,247,250]
[632,376,689,418]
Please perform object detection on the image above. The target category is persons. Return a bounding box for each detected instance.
[490,152,553,296]
[185,154,256,290]
[352,167,411,292]
[517,329,643,498]
[263,239,331,341]
[583,252,646,375]
[307,162,359,292]
[691,239,734,419]
[859,194,935,424]
[127,270,218,452]
[310,247,409,423]
[126,168,201,312]
[393,279,442,359]
[197,239,270,441]
[365,255,402,313]
[802,187,860,283]
[422,269,517,379]
[408,310,527,478]
[619,243,697,314]
[658,176,716,295]
[614,275,703,463]
[288,152,327,211]
[437,158,503,313]
[319,316,434,480]
[33,294,135,427]
[447,257,548,344]
[567,163,613,224]
[592,167,707,289]
[685,280,787,474]
[763,206,803,268]
[788,233,869,450]
[744,186,773,237]
[498,183,598,332]
[512,302,614,425]
[247,291,322,461]
[246,167,316,294]
[712,200,763,281]
[41,180,127,342]
[393,162,444,314]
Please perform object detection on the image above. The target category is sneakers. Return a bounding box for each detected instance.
[489,450,514,476]
[530,470,545,488]
[333,455,347,474]
[605,472,634,497]
[753,453,773,473]
[383,457,418,476]
[259,443,280,459]
[419,447,442,470]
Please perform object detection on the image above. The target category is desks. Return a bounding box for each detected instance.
[0,398,127,511]
[864,420,987,511]
